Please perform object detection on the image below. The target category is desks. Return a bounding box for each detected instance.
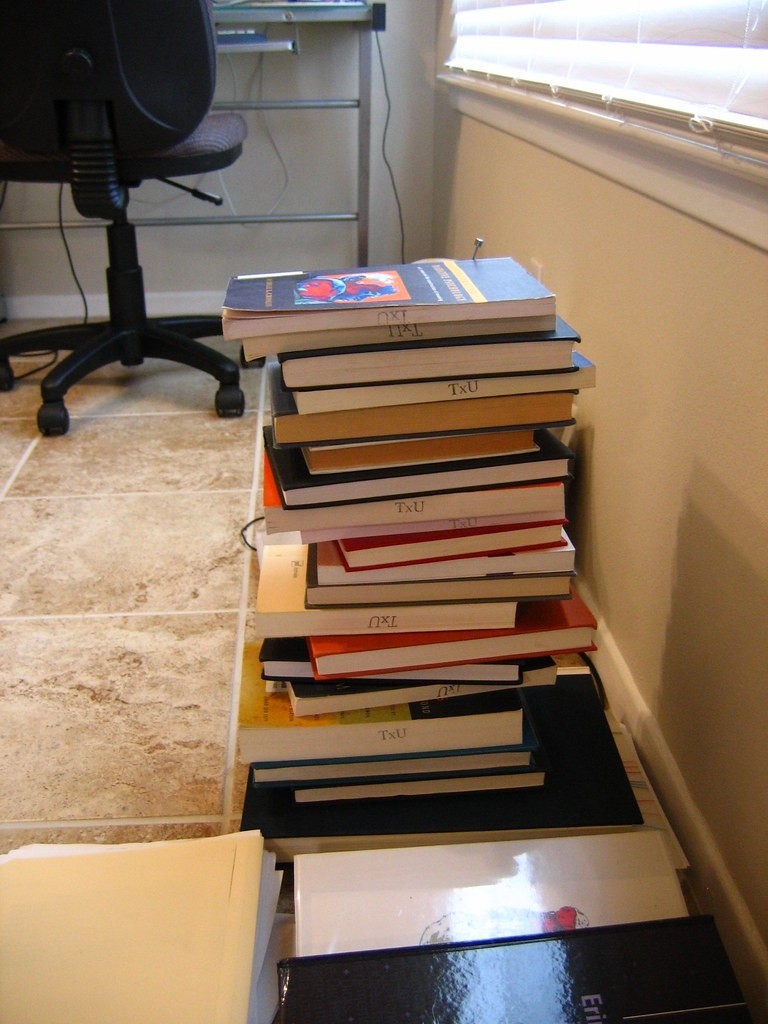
[1,0,387,266]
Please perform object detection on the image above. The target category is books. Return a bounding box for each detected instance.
[227,251,649,860]
[4,829,282,1024]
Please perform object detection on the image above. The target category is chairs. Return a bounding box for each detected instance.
[1,0,266,438]
